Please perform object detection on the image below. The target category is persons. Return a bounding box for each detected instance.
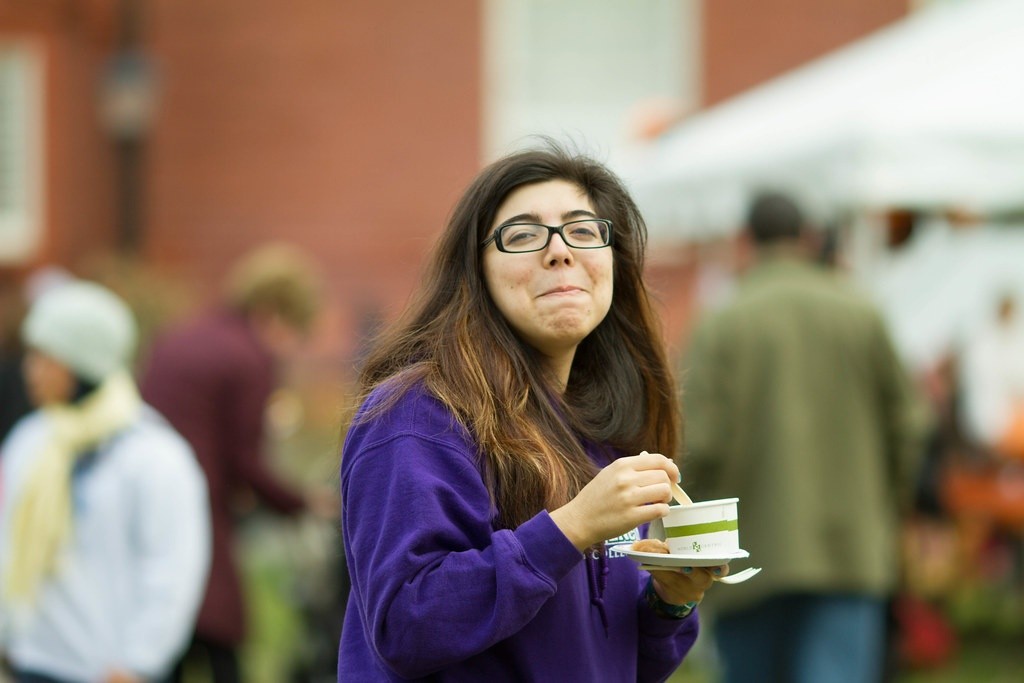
[679,193,1024,683]
[0,244,346,683]
[337,141,731,683]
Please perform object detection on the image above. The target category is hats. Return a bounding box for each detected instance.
[24,279,138,386]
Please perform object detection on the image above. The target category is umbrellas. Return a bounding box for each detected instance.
[619,2,1023,248]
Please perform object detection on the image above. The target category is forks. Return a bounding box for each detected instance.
[637,565,762,584]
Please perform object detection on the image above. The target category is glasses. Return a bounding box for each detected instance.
[480,219,613,253]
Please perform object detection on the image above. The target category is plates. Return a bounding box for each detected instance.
[610,545,750,567]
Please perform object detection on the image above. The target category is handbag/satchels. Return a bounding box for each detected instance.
[893,568,950,673]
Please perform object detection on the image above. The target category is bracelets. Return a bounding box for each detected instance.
[643,575,695,619]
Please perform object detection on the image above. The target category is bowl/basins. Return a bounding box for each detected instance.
[662,498,739,554]
